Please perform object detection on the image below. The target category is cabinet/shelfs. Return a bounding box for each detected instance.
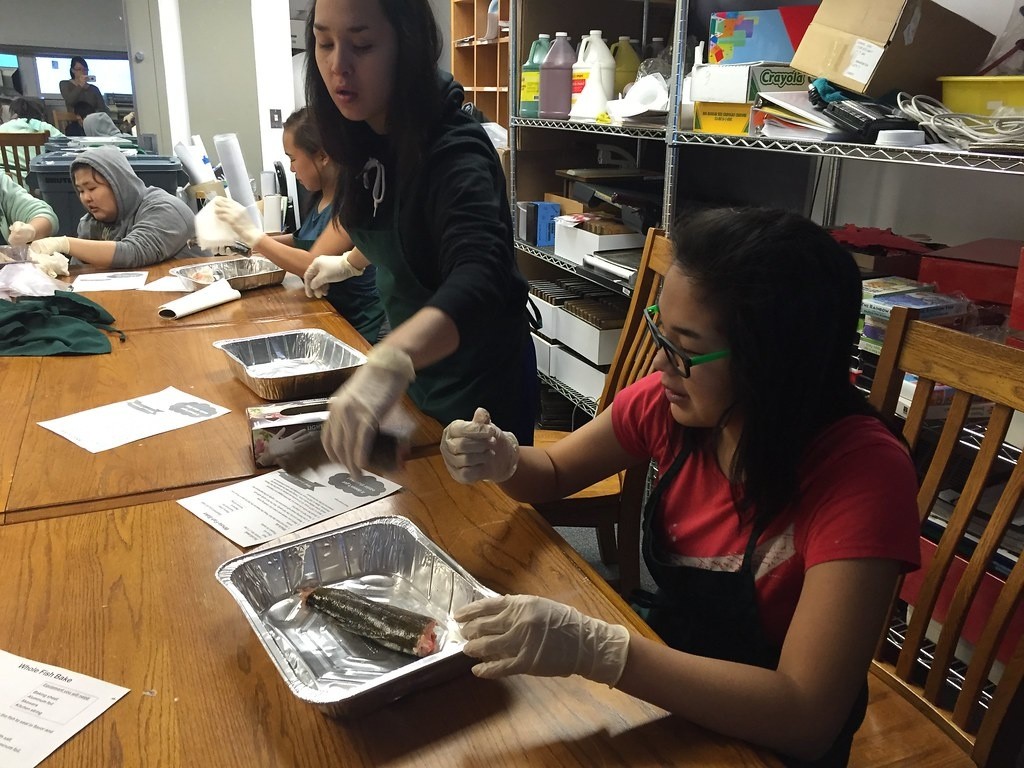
[508,0,1024,711]
[450,0,642,282]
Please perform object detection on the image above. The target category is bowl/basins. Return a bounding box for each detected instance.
[938,75,1024,133]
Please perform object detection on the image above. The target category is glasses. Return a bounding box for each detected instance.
[639,302,740,377]
[73,67,89,72]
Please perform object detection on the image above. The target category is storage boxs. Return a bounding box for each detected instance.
[28,136,182,240]
[521,0,1024,686]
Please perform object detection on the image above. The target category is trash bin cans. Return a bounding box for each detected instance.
[25,150,192,266]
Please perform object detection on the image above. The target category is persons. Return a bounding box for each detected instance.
[82,112,123,137]
[0,170,59,248]
[303,0,542,480]
[215,105,391,349]
[441,206,923,768]
[65,101,96,136]
[30,145,213,269]
[0,97,66,191]
[59,56,111,118]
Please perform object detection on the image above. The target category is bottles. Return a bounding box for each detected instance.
[518,29,664,119]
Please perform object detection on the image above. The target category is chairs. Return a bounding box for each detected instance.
[843,308,1023,768]
[533,227,673,610]
[1,110,81,193]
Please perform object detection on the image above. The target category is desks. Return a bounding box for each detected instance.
[1,258,787,768]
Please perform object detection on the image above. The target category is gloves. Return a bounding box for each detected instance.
[441,406,518,486]
[29,234,71,256]
[213,198,264,248]
[324,343,417,479]
[8,221,35,248]
[302,250,365,300]
[453,591,631,690]
